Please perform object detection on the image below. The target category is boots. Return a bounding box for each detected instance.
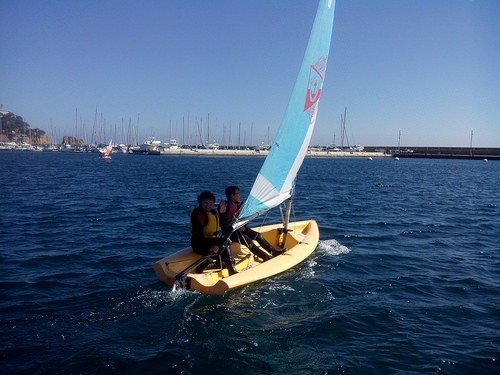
[222,247,239,276]
[196,262,225,274]
[249,242,272,261]
[256,235,287,257]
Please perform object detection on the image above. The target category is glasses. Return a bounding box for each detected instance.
[235,193,240,194]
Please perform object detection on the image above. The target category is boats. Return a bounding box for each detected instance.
[130,142,160,155]
[98,140,113,160]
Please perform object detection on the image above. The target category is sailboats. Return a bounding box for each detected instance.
[151,0,337,296]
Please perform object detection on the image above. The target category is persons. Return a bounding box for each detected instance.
[192,192,239,276]
[218,186,288,262]
